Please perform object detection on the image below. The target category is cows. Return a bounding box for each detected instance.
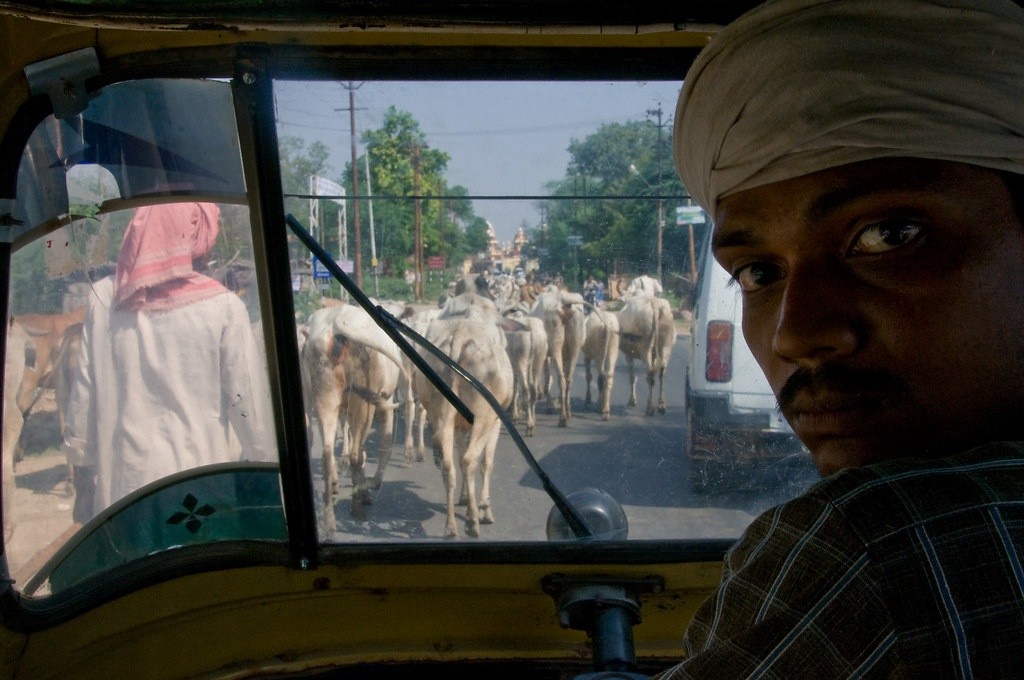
[618,275,677,416]
[3,291,620,544]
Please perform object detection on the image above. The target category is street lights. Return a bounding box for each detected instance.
[627,165,663,287]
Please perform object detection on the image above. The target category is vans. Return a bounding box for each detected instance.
[683,215,812,499]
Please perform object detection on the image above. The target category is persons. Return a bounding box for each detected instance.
[475,270,495,300]
[654,0,1024,680]
[521,256,527,266]
[63,188,277,557]
[583,274,605,314]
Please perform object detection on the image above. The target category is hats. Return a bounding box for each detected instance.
[672,0,1024,220]
